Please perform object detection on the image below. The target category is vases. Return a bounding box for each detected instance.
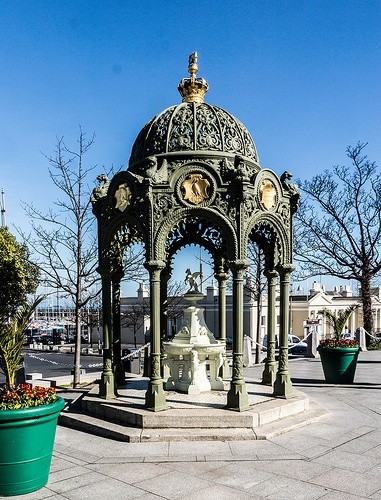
[316,347,362,385]
[0,397,65,496]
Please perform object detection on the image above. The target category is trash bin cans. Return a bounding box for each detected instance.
[121,348,142,376]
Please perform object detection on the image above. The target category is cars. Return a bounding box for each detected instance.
[263,335,307,355]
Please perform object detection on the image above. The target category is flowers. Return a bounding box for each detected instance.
[317,303,362,349]
[0,294,57,411]
